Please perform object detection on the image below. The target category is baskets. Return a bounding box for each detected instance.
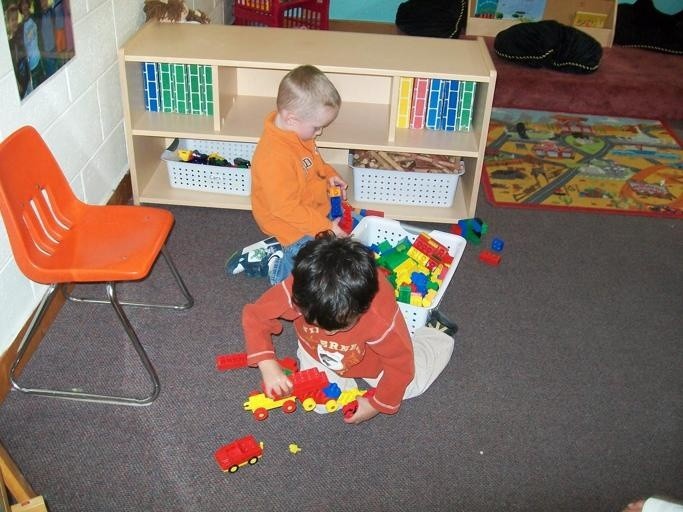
[348,149,466,207]
[346,215,467,338]
[160,138,258,196]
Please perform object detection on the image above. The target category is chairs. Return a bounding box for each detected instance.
[0,125,193,409]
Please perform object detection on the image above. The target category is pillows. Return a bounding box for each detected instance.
[493,18,603,74]
[396,0,465,39]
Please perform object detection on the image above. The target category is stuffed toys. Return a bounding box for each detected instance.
[143,0,211,25]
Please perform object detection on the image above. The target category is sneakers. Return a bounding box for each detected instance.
[427,310,457,335]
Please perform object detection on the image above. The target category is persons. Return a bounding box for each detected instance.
[242,228,458,428]
[223,64,348,288]
[5,0,75,101]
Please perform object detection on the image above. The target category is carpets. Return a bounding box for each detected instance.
[483,106,683,217]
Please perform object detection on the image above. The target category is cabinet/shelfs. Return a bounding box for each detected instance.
[117,17,498,225]
